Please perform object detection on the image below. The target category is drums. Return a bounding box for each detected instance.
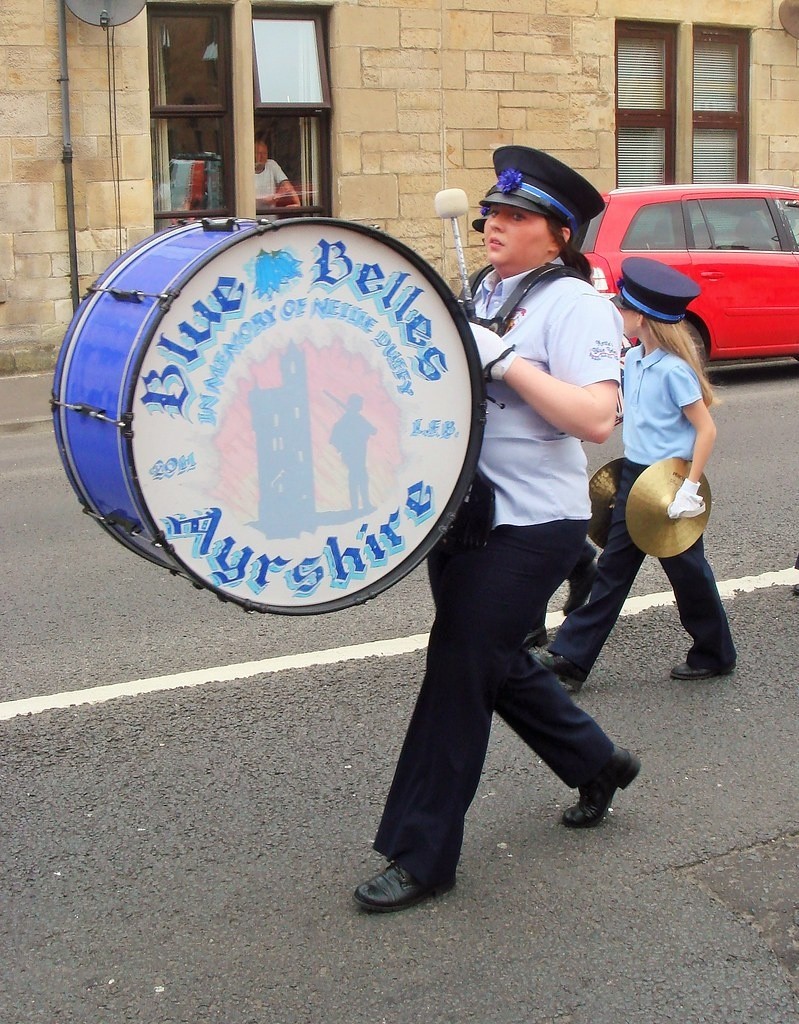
[48,213,489,618]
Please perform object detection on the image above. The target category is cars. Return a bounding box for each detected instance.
[573,182,798,371]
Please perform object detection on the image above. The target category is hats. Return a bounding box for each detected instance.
[479,145,606,237]
[609,257,702,324]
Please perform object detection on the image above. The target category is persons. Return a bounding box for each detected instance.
[353,143,643,915]
[250,135,301,221]
[533,257,737,695]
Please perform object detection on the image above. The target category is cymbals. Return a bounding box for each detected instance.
[625,455,713,557]
[588,456,628,550]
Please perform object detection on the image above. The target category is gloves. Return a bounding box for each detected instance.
[467,321,518,380]
[668,479,705,519]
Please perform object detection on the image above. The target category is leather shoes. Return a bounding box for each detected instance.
[563,748,642,828]
[528,648,588,688]
[669,657,738,680]
[562,564,600,615]
[352,861,455,913]
